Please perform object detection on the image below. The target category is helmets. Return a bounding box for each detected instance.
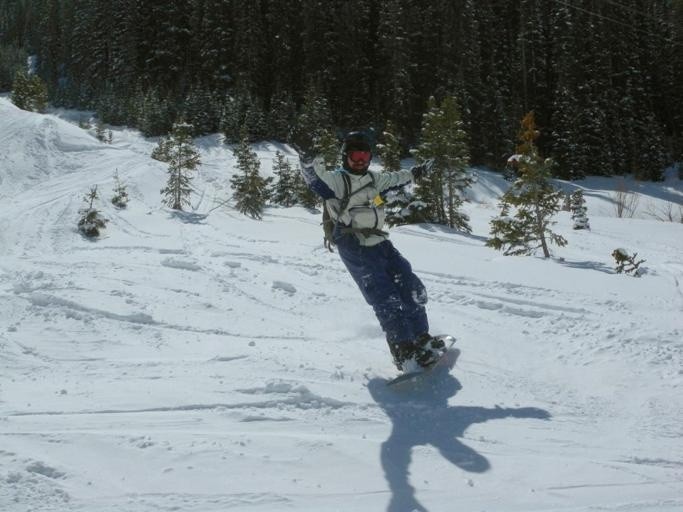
[341,131,373,155]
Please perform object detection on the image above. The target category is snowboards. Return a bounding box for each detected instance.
[386,334,455,388]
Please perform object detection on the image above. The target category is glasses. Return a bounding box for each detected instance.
[349,151,372,163]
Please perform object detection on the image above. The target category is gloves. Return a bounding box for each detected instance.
[412,159,434,178]
[288,126,316,167]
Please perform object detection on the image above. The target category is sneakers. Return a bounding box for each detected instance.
[418,334,448,350]
[395,350,440,370]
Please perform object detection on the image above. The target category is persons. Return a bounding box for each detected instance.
[289,130,447,371]
